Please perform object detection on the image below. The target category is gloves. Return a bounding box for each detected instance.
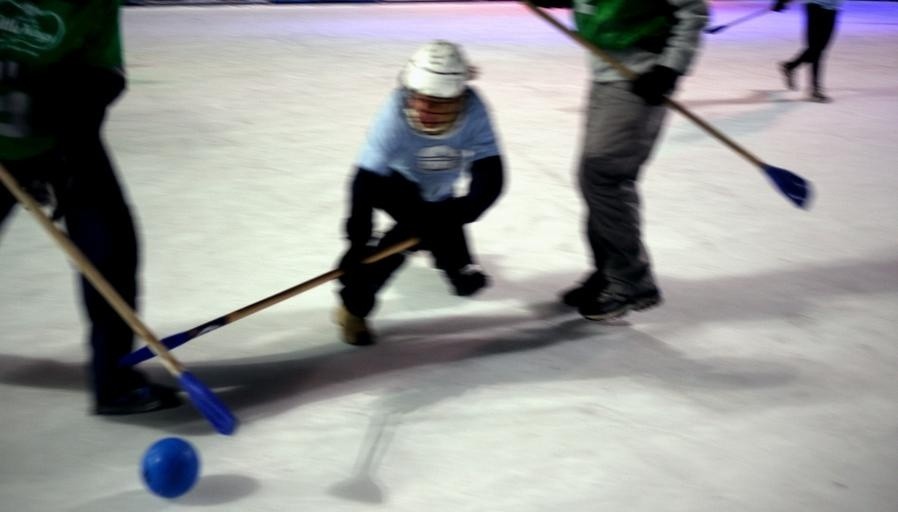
[627,63,682,105]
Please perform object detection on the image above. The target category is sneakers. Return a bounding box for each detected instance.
[560,269,666,323]
[776,59,799,91]
[807,88,835,104]
[332,304,381,346]
[89,367,186,417]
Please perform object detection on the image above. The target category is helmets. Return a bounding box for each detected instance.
[398,39,473,100]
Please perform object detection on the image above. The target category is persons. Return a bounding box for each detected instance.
[523,0,710,324]
[766,0,845,101]
[1,0,189,419]
[328,38,508,348]
